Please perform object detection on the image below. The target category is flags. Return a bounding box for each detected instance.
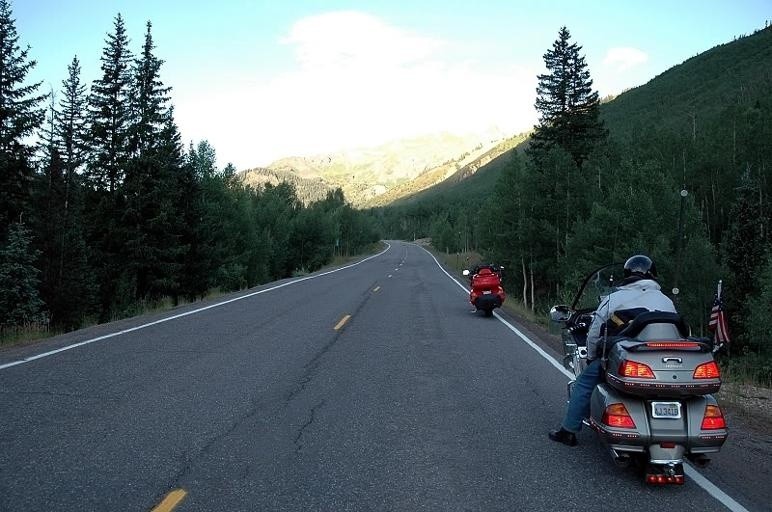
[708,282,732,350]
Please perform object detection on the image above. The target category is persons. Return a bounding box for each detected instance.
[549,255,678,446]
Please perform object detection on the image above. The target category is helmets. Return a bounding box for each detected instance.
[623,254,658,279]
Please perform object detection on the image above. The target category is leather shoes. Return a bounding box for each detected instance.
[548,428,577,447]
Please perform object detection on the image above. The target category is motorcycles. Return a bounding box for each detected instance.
[549,261,731,485]
[462,261,507,318]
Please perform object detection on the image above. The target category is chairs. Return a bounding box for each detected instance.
[476,266,493,274]
[614,310,691,342]
